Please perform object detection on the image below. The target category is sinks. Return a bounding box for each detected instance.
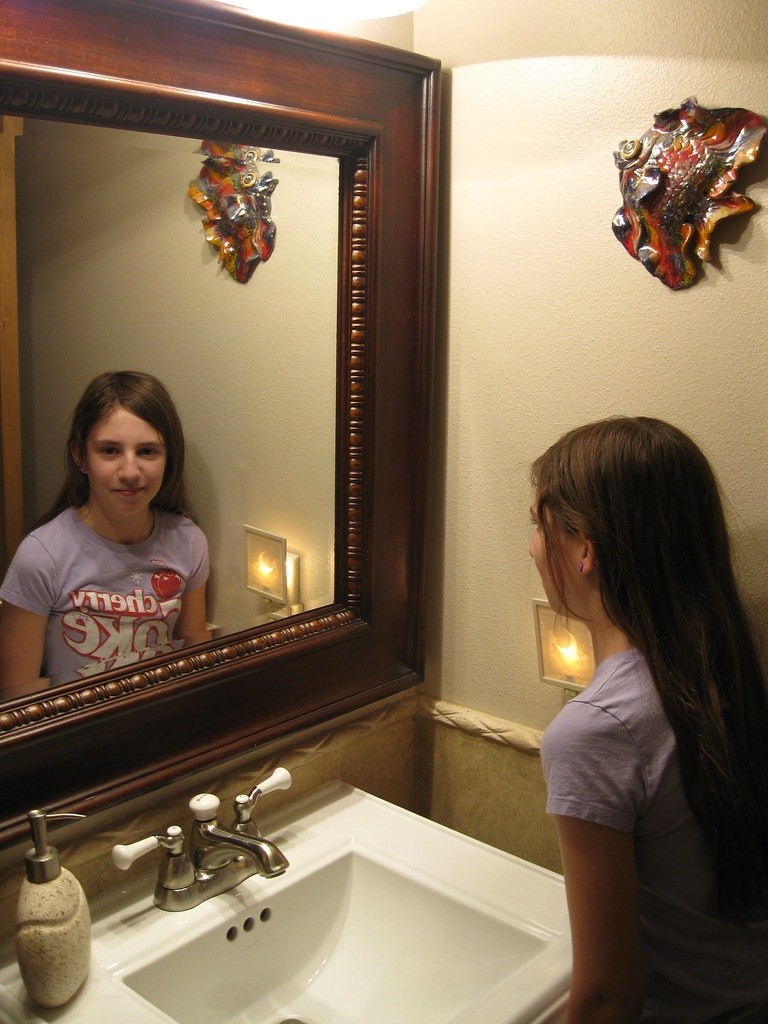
[105,847,553,1024]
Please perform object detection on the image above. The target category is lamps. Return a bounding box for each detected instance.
[237,519,302,620]
[528,598,597,696]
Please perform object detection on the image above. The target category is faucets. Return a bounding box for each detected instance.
[111,766,293,913]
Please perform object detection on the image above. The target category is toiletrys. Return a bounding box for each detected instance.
[14,809,92,1010]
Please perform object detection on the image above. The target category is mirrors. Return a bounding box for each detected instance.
[0,1,442,843]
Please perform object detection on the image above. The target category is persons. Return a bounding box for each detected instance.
[528,417,768,1024]
[0,369,209,688]
